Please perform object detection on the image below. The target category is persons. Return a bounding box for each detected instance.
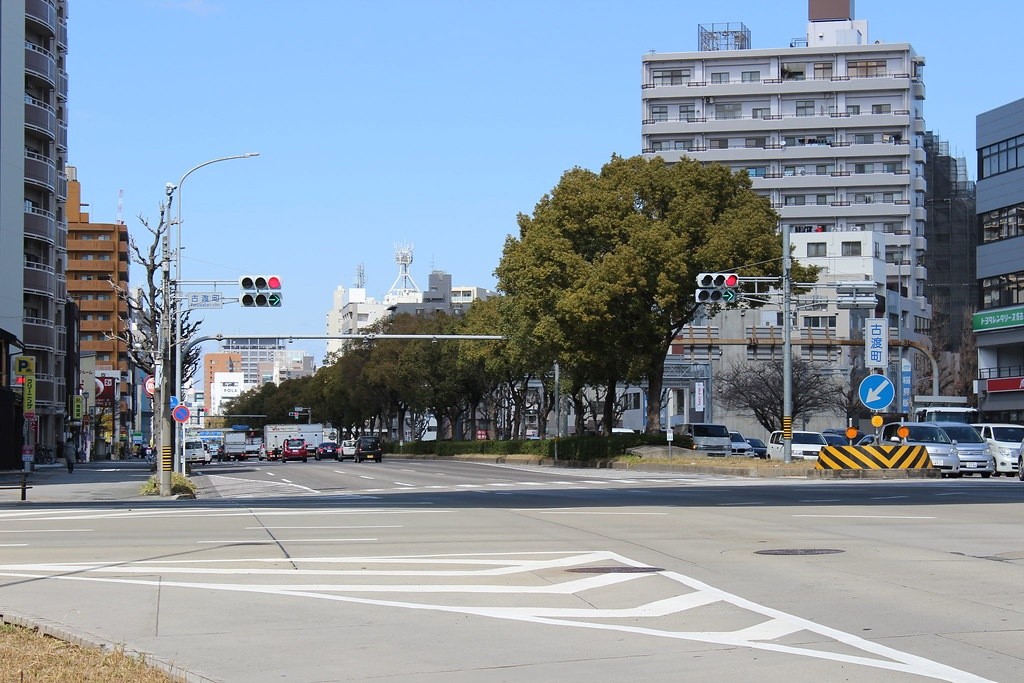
[64,437,76,474]
[145,445,153,456]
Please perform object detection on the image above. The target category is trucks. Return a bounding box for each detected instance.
[271,424,337,461]
[222,431,247,461]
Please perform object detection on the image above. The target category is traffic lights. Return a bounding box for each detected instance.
[696,290,735,302]
[239,275,281,291]
[239,291,282,307]
[698,273,740,288]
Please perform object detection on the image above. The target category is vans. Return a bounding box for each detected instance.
[765,431,832,461]
[674,423,733,457]
[970,423,1024,477]
[597,426,635,434]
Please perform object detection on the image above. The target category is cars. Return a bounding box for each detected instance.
[315,436,382,463]
[726,430,764,459]
[879,422,961,477]
[183,438,220,466]
[822,428,878,448]
[926,421,994,481]
[257,438,308,463]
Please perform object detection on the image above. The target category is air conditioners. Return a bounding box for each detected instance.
[851,227,861,231]
[704,97,714,103]
[831,228,841,232]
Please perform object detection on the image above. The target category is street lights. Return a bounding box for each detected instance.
[175,153,261,466]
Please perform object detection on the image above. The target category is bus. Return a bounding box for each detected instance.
[915,408,980,424]
[246,430,263,453]
